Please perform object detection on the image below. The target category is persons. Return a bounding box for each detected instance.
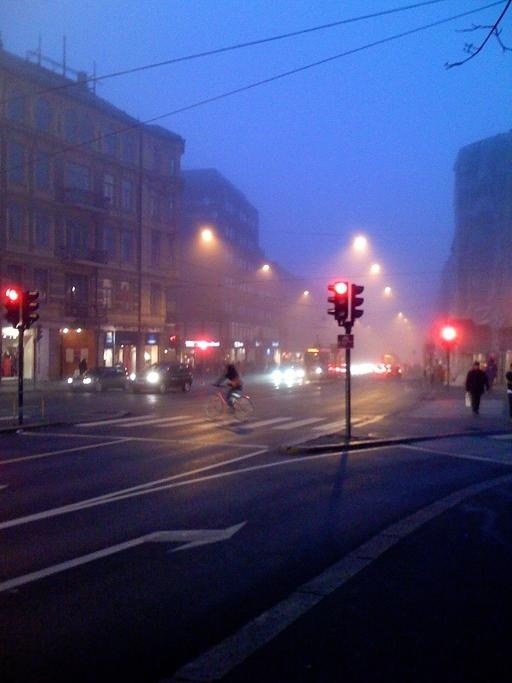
[79,359,87,374]
[215,363,241,406]
[466,357,512,422]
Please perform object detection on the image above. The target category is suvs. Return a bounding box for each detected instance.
[130,362,194,394]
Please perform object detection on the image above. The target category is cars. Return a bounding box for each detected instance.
[372,354,410,378]
[67,365,130,394]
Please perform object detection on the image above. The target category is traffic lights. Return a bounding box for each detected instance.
[170,334,177,348]
[4,287,18,322]
[326,284,334,316]
[354,284,364,318]
[441,326,457,341]
[23,289,40,321]
[334,281,347,320]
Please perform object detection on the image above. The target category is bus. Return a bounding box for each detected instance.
[303,346,346,381]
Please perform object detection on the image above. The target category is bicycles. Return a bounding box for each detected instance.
[205,384,256,422]
